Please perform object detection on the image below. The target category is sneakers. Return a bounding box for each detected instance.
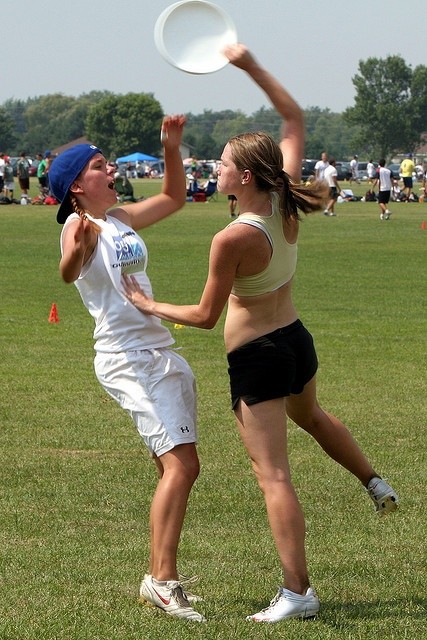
[246,583,320,623]
[366,477,399,511]
[139,573,205,603]
[143,575,207,623]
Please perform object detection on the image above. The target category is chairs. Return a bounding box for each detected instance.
[205,181,217,202]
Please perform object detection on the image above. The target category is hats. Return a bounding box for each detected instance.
[48,144,102,224]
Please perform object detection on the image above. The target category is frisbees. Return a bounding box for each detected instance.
[154,0,237,74]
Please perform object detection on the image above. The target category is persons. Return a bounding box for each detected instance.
[324,160,341,216]
[181,157,221,198]
[121,45,400,622]
[314,151,330,179]
[371,159,394,220]
[16,152,31,195]
[136,159,158,179]
[35,154,49,196]
[4,155,15,201]
[349,154,361,185]
[367,159,377,183]
[48,115,207,622]
[415,164,427,197]
[114,172,133,196]
[44,151,56,174]
[398,153,416,198]
[0,154,4,193]
[126,161,133,178]
[391,180,404,202]
